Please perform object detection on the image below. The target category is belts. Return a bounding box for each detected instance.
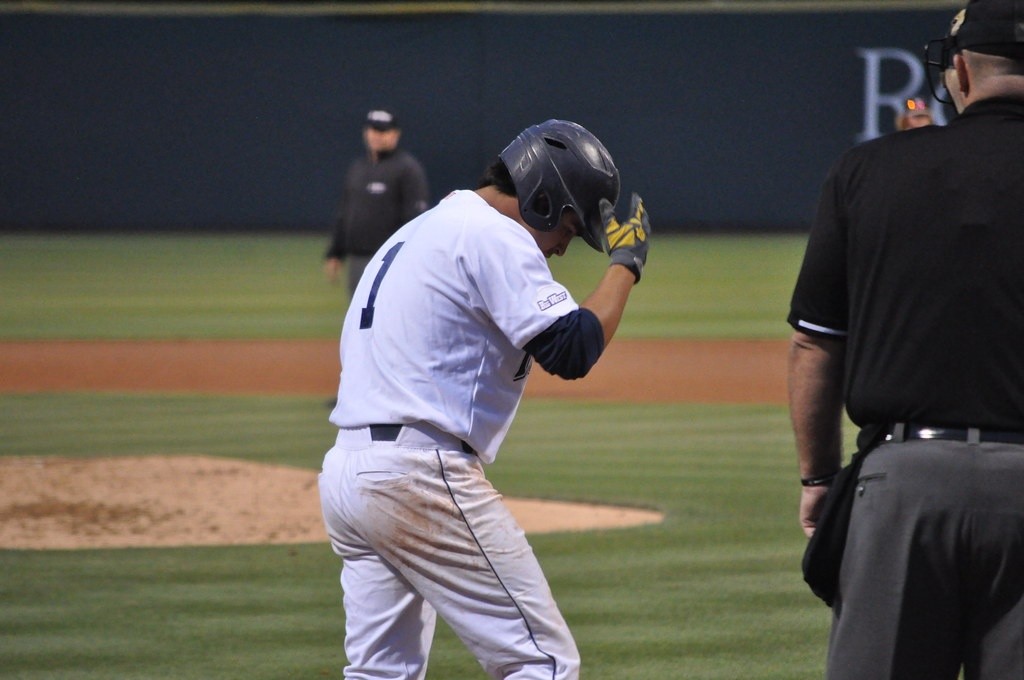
[369,424,474,454]
[876,423,1024,444]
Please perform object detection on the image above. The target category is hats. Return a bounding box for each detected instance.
[905,97,930,116]
[366,106,399,131]
[950,0,1024,60]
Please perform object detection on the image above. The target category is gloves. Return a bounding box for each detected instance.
[599,193,651,285]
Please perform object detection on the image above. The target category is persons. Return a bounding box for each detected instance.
[318,118,651,680]
[785,0,1024,680]
[325,108,430,299]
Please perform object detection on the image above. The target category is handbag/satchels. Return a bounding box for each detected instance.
[801,424,885,608]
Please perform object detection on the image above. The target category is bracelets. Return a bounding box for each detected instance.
[801,468,840,486]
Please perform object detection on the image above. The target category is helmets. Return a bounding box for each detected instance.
[499,120,620,253]
